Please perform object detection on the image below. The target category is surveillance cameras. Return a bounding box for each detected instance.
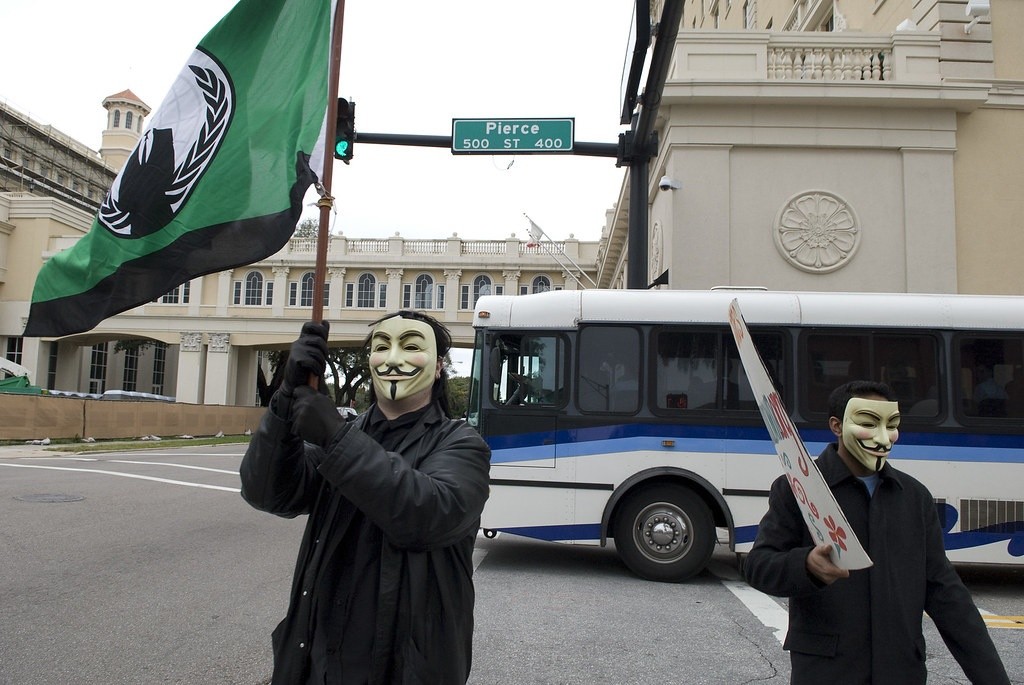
[659,176,671,191]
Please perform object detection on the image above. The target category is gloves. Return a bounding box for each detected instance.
[280,320,330,396]
[288,386,346,454]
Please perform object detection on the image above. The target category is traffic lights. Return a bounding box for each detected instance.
[331,97,356,165]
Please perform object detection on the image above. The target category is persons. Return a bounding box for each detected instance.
[240,310,492,685]
[744,381,1013,685]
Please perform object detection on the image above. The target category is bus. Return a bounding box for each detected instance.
[464,289,1024,583]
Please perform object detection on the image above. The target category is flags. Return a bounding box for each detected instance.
[22,0,333,337]
[527,222,544,247]
